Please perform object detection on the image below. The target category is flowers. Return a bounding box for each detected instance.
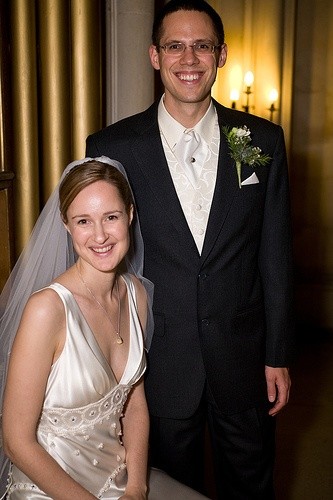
[219,124,272,168]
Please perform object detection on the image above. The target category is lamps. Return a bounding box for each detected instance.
[230,71,279,122]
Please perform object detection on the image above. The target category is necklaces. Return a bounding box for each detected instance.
[74,262,123,344]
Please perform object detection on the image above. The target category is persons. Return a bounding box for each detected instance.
[85,0,295,500]
[0,156,211,500]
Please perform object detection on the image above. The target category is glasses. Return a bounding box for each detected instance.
[159,42,220,54]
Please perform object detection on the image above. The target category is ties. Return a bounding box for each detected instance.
[176,131,199,189]
